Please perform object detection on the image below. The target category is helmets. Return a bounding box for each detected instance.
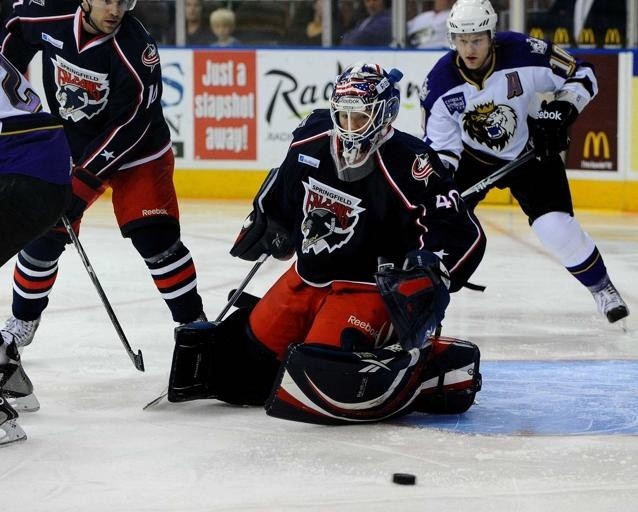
[446,0,499,39]
[329,62,400,153]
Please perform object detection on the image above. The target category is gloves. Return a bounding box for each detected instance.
[53,165,104,231]
[529,99,578,155]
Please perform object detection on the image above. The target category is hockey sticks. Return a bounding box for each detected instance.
[61,214,144,371]
[142,253,268,409]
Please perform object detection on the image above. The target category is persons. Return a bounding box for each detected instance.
[128,0,638,53]
[0,0,208,426]
[207,61,489,426]
[420,0,632,323]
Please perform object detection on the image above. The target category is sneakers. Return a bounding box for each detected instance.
[0,314,39,349]
[590,281,631,324]
[0,339,33,425]
